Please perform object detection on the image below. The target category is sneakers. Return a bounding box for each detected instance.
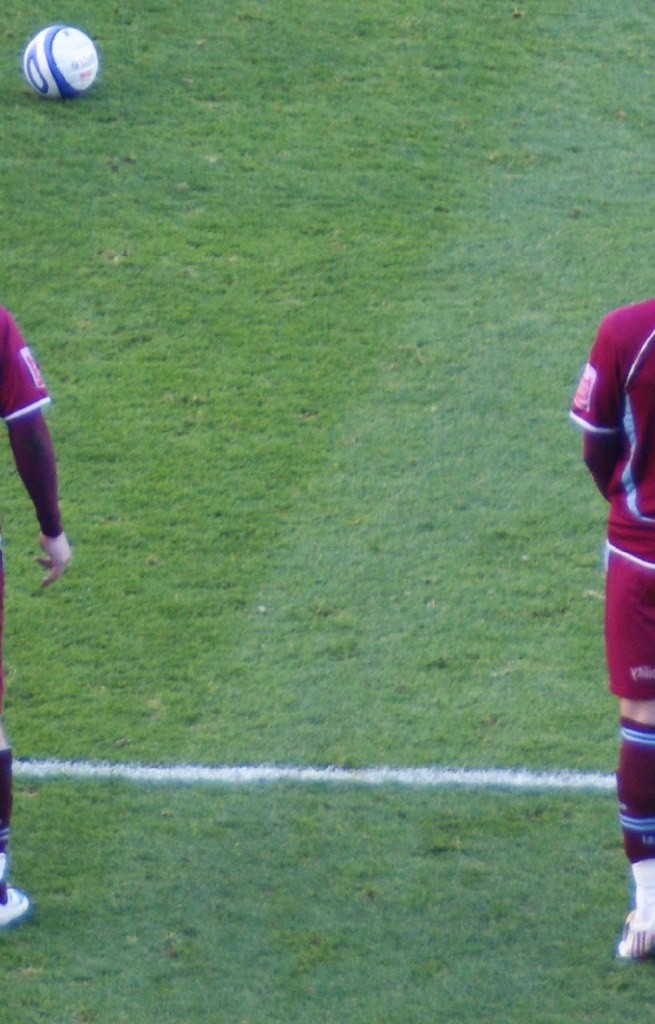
[0,889,29,925]
[616,911,655,958]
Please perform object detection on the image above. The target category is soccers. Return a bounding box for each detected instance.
[20,22,101,102]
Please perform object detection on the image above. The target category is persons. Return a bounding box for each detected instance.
[569,299,655,959]
[0,303,72,926]
